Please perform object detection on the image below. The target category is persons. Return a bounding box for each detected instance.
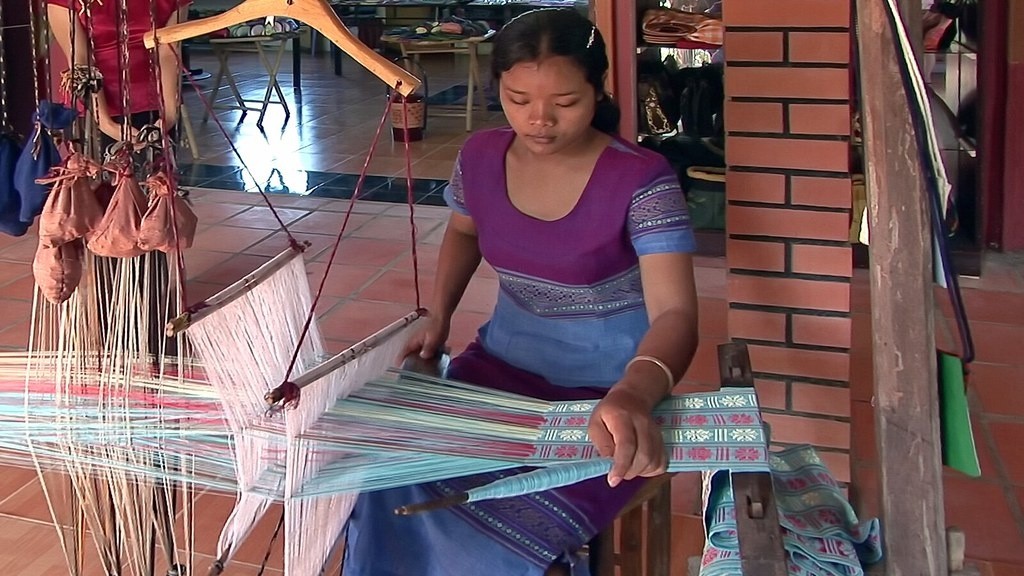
[45,0,194,355]
[341,11,700,576]
[922,0,957,53]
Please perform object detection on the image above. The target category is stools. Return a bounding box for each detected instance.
[601,471,672,576]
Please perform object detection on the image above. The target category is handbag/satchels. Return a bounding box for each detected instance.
[637,9,726,193]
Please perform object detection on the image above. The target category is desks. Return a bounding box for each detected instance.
[381,30,497,135]
[325,0,457,75]
[201,28,307,129]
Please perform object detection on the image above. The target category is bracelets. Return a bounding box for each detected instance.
[624,355,674,398]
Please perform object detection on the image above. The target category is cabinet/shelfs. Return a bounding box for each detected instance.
[924,42,977,152]
